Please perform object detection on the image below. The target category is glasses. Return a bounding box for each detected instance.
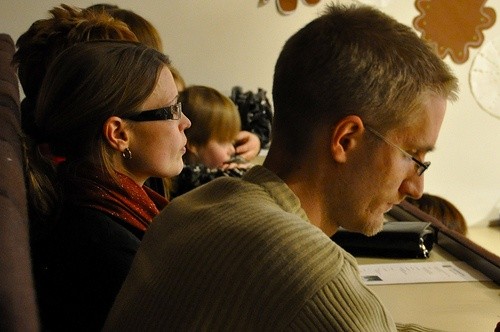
[366,126,431,176]
[119,102,182,122]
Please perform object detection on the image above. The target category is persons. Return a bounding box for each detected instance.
[15,3,187,202]
[169,86,270,202]
[29,39,191,332]
[405,193,467,240]
[104,6,455,332]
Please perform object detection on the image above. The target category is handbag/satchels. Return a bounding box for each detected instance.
[329,221,435,261]
